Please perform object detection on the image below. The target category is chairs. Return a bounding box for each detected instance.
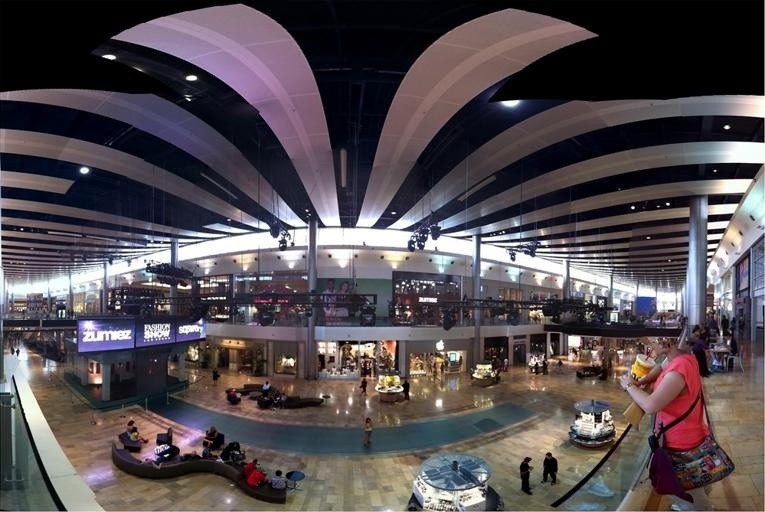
[706,328,747,375]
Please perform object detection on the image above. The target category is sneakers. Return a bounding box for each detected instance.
[670,502,714,511]
[522,480,555,495]
[589,479,615,497]
[571,502,607,510]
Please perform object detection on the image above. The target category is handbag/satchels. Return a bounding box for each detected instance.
[668,436,734,490]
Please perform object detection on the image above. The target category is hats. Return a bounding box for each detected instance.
[650,449,694,504]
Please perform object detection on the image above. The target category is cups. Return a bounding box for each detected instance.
[629,353,656,382]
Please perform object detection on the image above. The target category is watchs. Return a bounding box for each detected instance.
[624,382,635,392]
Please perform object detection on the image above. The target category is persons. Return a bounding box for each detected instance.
[689,315,746,378]
[432,353,563,383]
[520,457,534,495]
[126,420,286,490]
[212,367,218,384]
[230,381,287,416]
[335,281,351,326]
[362,417,373,448]
[8,332,22,358]
[540,452,557,485]
[402,380,410,400]
[359,379,368,396]
[320,278,336,326]
[619,308,715,511]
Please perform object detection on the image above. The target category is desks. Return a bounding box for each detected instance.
[285,470,306,494]
[322,394,331,406]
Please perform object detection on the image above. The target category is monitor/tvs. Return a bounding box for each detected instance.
[76,318,134,351]
[136,316,175,348]
[176,313,206,343]
[450,352,460,361]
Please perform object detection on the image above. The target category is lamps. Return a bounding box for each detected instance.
[253,119,295,253]
[503,152,544,260]
[405,163,444,253]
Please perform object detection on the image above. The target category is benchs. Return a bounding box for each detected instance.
[224,381,324,410]
[106,429,287,505]
[577,366,609,381]
